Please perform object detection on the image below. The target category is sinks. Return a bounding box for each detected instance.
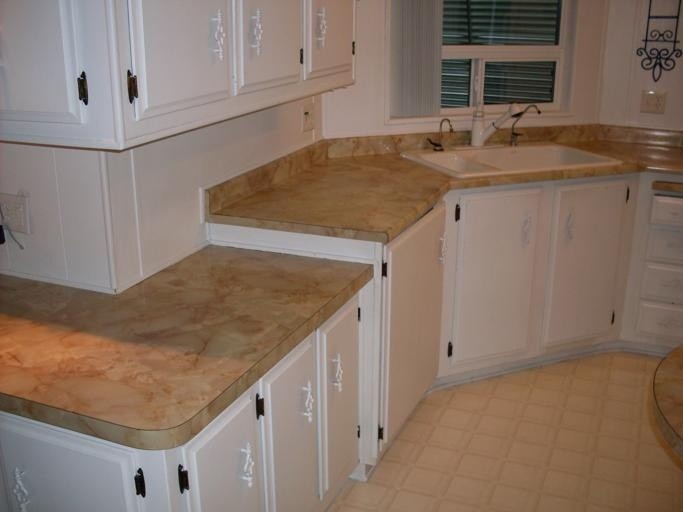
[461,138,624,173]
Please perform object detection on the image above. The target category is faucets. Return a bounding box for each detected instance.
[399,144,501,179]
[509,104,542,147]
[471,102,525,147]
[426,118,455,151]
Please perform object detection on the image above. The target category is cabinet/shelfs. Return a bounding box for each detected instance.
[256,294,361,511]
[633,193,683,340]
[305,1,356,82]
[0,2,90,126]
[0,420,147,512]
[125,2,302,121]
[445,184,631,366]
[362,208,439,484]
[177,392,267,512]
